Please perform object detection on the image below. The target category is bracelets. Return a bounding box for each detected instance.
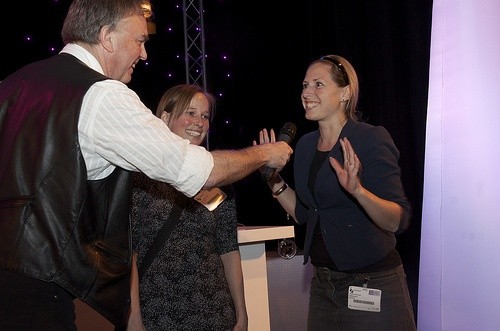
[273,183,288,197]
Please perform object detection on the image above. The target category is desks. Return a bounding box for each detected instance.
[236,224,295,331]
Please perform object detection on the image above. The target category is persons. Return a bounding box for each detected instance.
[253,54,416,331]
[127,85,248,331]
[0,0,293,331]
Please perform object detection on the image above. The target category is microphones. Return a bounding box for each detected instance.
[263,122,296,182]
